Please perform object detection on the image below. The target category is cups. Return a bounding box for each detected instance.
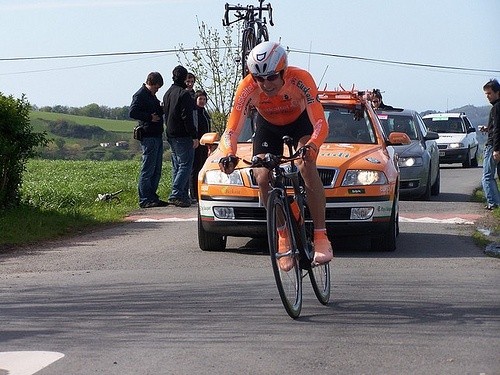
[481,128,487,136]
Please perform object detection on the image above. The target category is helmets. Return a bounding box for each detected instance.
[247,41,288,75]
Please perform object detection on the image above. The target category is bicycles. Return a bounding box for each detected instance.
[225,136,333,320]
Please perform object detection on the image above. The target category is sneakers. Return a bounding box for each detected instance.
[278,237,294,270]
[314,240,332,263]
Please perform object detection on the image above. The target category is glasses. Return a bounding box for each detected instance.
[257,72,282,82]
[329,123,343,129]
[372,101,378,103]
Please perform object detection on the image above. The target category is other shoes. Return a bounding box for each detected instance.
[485,205,497,210]
[144,200,167,208]
[168,199,190,207]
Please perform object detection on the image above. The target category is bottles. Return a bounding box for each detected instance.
[287,195,304,225]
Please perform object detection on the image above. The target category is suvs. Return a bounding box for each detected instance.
[421,112,480,168]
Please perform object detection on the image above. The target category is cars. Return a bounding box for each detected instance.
[325,110,441,202]
[197,90,412,252]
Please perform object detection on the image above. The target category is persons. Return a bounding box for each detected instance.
[185,72,211,202]
[327,111,353,137]
[162,64,193,207]
[393,118,411,133]
[218,41,331,271]
[129,72,168,207]
[479,80,500,209]
